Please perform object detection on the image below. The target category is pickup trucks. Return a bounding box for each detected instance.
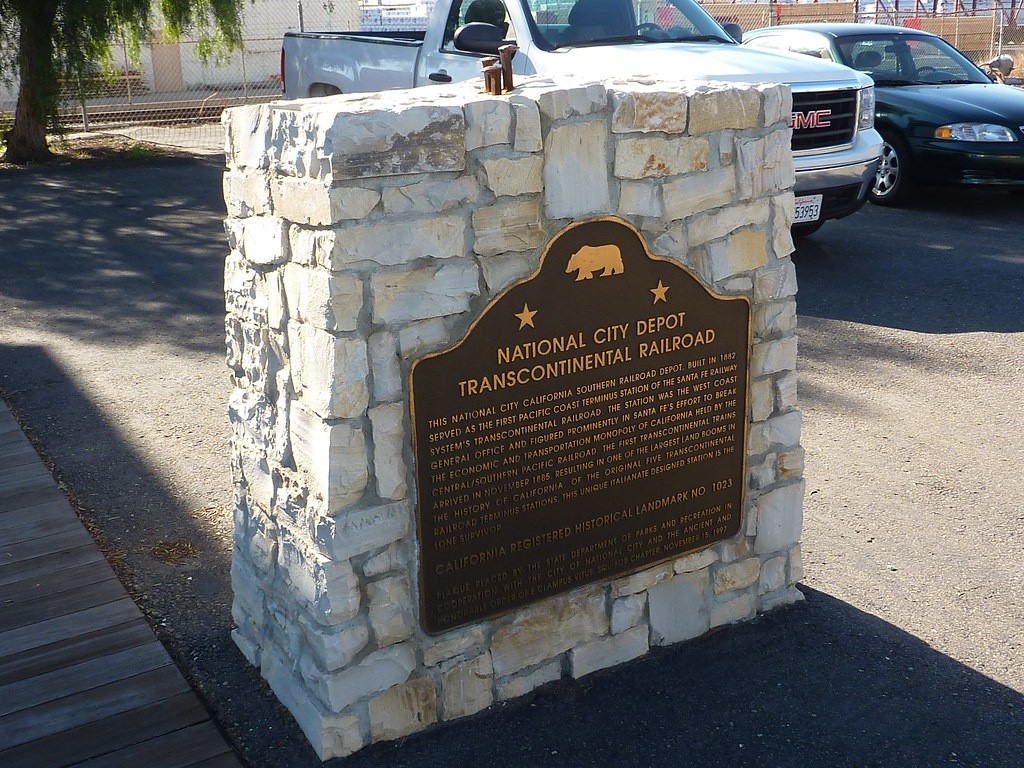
[281,0,885,242]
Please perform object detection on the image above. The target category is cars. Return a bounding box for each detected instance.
[739,21,1024,203]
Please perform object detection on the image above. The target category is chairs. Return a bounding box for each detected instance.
[565,0,637,39]
[854,51,888,82]
[465,0,509,39]
